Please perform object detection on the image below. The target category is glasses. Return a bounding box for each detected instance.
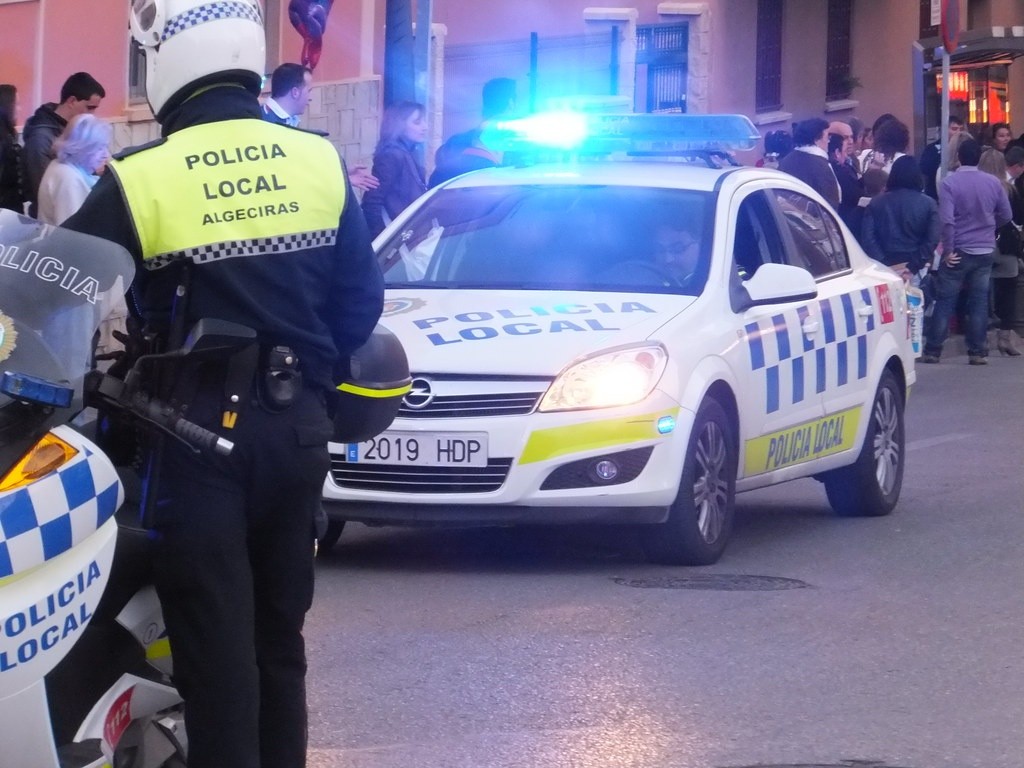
[653,238,697,254]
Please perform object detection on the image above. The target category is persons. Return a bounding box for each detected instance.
[619,205,701,289]
[427,121,504,191]
[0,83,24,215]
[434,76,518,167]
[752,113,1024,364]
[35,114,114,227]
[358,99,427,242]
[259,62,381,191]
[58,2,387,767]
[22,71,105,221]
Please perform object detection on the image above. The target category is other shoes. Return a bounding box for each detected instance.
[969,356,988,365]
[916,354,941,363]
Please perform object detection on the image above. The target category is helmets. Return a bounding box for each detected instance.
[130,1,265,121]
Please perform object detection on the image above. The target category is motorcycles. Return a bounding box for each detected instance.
[2,202,300,761]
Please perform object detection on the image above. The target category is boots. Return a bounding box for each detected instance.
[997,329,1022,357]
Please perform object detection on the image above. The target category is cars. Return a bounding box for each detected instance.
[320,107,926,565]
